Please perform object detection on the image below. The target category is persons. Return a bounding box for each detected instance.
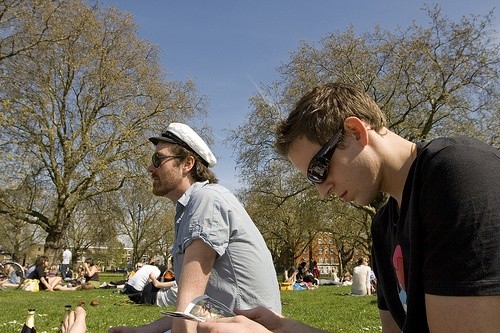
[61,307,87,333]
[0,246,378,305]
[108,123,282,333]
[160,83,500,333]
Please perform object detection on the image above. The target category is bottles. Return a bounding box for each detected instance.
[61,305,72,327]
[20,308,36,333]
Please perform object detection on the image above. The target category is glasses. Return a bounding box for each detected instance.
[307,128,344,186]
[152,153,186,168]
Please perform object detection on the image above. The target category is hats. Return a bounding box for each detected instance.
[148,122,217,168]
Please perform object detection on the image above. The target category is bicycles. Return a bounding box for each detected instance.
[0,249,25,291]
